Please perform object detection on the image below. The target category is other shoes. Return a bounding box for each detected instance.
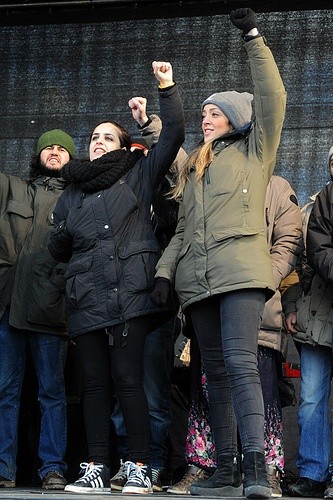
[0,477,15,488]
[288,476,326,497]
[268,478,282,497]
[41,471,67,489]
[167,468,210,494]
[324,482,333,500]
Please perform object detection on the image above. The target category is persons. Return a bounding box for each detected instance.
[0,6,333,500]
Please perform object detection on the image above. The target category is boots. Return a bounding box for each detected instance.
[243,452,271,498]
[190,453,243,497]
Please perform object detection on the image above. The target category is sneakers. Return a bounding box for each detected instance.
[150,469,163,492]
[64,462,111,495]
[122,464,153,495]
[110,459,135,490]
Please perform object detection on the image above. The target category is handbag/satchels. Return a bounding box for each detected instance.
[278,379,297,408]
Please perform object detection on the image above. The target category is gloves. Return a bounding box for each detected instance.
[229,8,259,34]
[149,277,170,307]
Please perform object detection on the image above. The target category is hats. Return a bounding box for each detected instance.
[201,90,254,132]
[128,133,149,152]
[327,145,333,171]
[36,129,75,160]
